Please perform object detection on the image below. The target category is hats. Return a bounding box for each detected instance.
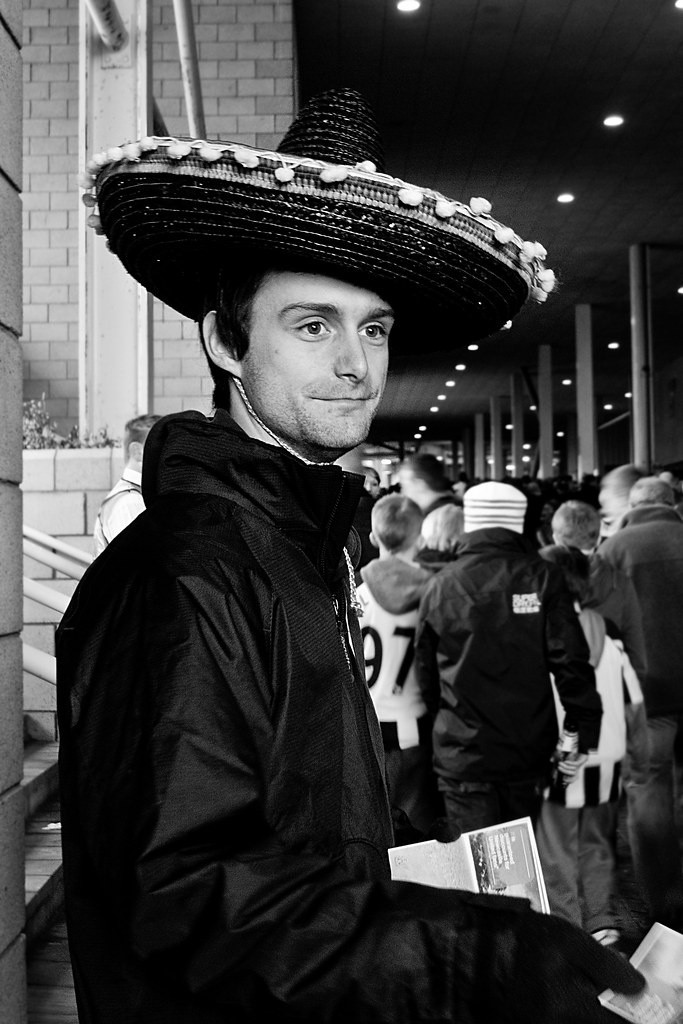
[82,87,554,349]
[463,481,528,534]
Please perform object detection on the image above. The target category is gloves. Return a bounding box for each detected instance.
[513,906,645,1024]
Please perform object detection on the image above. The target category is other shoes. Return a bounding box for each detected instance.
[589,929,621,947]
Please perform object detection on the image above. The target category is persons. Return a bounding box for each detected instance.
[54,88,647,1024]
[93,415,683,962]
